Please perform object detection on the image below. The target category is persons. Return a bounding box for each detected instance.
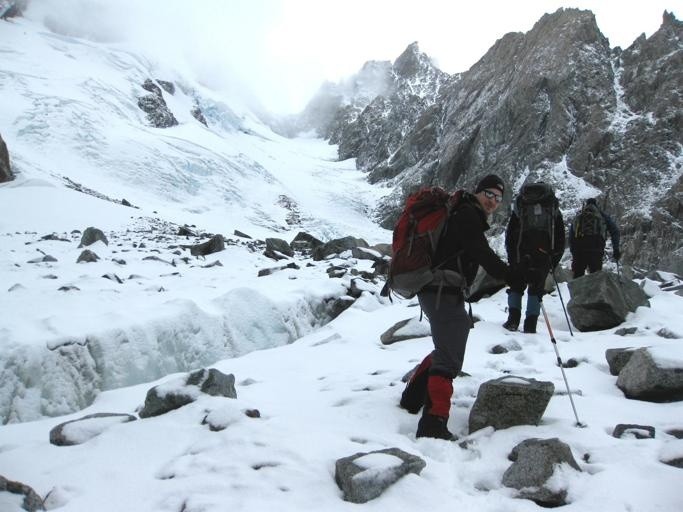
[502,177,567,338]
[400,173,532,451]
[567,197,622,280]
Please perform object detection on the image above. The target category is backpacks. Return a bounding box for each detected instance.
[573,205,607,248]
[514,181,558,243]
[379,185,452,305]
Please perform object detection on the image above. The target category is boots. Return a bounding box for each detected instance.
[400,381,427,414]
[503,308,521,331]
[523,315,537,333]
[416,415,457,441]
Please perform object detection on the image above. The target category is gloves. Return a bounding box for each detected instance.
[547,250,561,267]
[504,263,530,292]
[613,251,621,261]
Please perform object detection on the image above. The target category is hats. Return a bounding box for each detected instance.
[476,174,504,196]
